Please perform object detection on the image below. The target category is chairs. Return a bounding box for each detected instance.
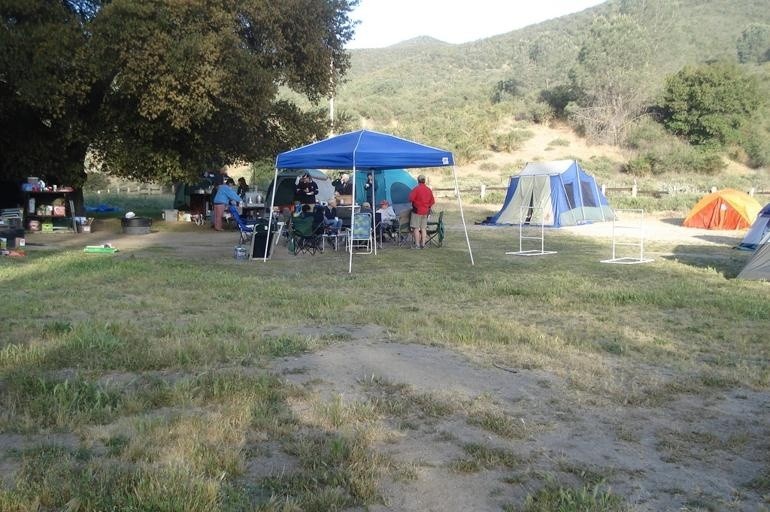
[207,196,445,264]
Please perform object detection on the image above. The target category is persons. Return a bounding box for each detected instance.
[408,174,434,254]
[281,171,397,245]
[212,178,243,231]
[237,177,249,198]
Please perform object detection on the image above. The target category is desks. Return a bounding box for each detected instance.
[24,189,80,235]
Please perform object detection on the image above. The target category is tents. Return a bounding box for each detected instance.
[681,188,770,283]
[479,157,619,227]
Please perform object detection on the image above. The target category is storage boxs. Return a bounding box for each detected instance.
[78,225,91,233]
[29,205,67,233]
[163,208,192,223]
